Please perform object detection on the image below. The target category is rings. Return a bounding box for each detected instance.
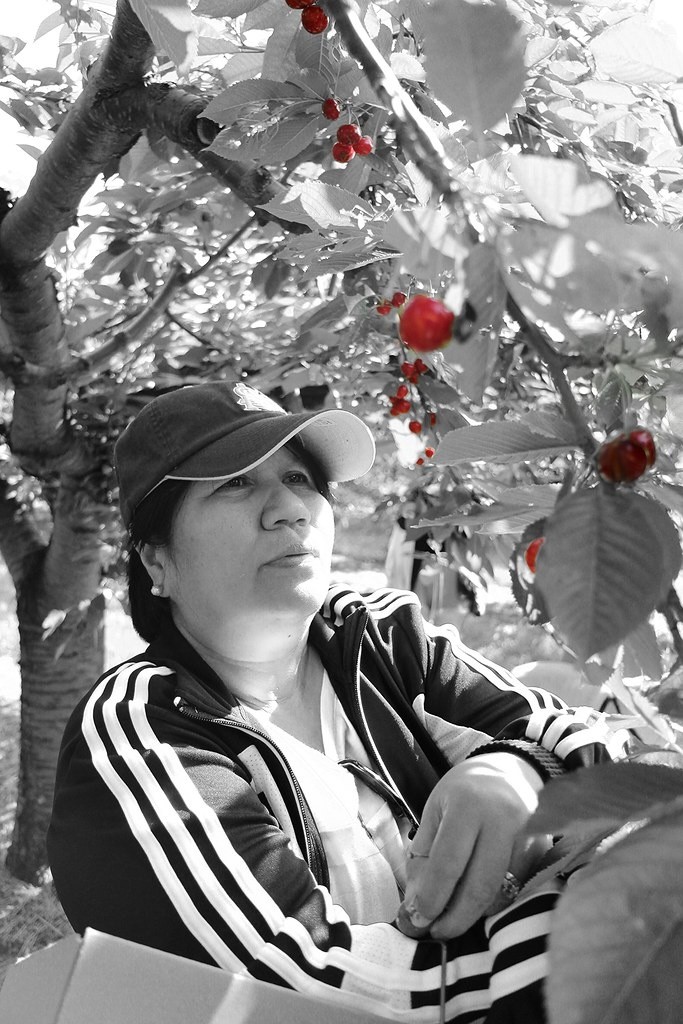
[500,871,520,898]
[407,849,429,859]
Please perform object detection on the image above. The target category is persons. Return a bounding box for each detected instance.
[386,511,484,614]
[43,384,643,1024]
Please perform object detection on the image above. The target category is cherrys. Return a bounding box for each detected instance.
[285,0,372,164]
[524,381,656,573]
[374,278,456,465]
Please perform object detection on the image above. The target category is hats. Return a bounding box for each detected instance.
[114,383,376,528]
